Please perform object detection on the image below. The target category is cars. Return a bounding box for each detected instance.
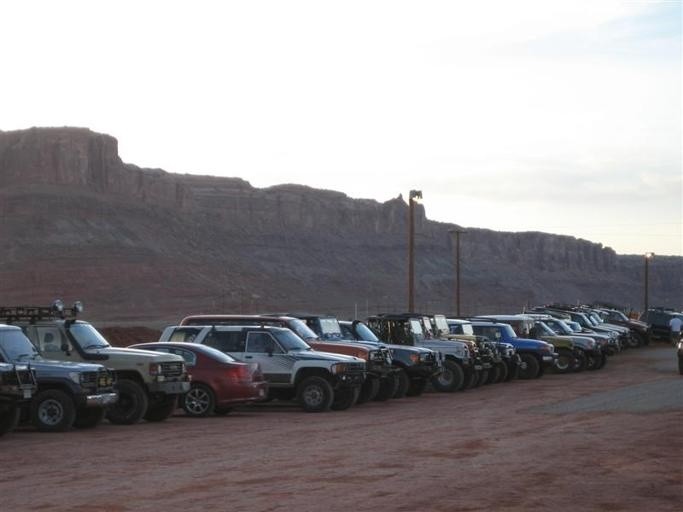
[124,342,265,419]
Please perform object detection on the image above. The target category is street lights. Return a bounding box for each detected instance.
[406,188,421,317]
[445,227,468,315]
[643,252,654,314]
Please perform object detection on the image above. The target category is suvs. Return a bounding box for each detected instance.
[156,322,367,415]
[1,301,194,427]
[364,303,651,391]
[638,307,683,344]
[299,316,446,399]
[0,324,120,431]
[179,314,394,405]
